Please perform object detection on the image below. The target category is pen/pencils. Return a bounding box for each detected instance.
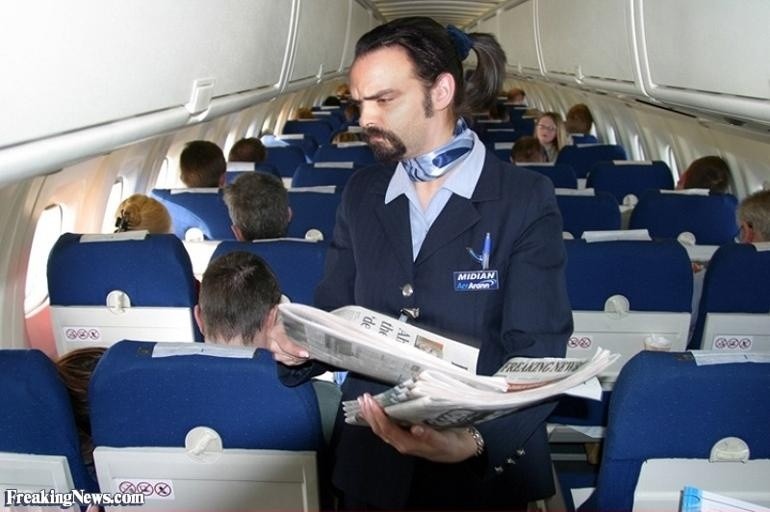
[483,233,491,271]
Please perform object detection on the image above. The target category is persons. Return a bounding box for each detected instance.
[487,101,510,121]
[227,171,293,242]
[260,127,288,148]
[462,422,484,457]
[262,16,575,512]
[46,346,163,512]
[295,107,313,119]
[565,104,598,145]
[181,139,227,189]
[676,156,731,197]
[113,192,172,234]
[336,83,350,99]
[228,137,266,167]
[323,96,341,106]
[507,88,525,104]
[331,131,361,145]
[343,102,361,121]
[509,135,545,163]
[193,251,282,345]
[691,189,770,350]
[534,112,565,163]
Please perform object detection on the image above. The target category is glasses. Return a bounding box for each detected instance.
[537,123,557,133]
[736,223,753,235]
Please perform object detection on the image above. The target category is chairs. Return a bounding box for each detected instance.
[312,141,374,167]
[89,339,329,512]
[546,228,693,445]
[627,189,739,263]
[311,110,343,132]
[470,95,542,162]
[555,143,627,178]
[341,122,361,132]
[554,187,622,240]
[585,159,675,208]
[225,161,282,186]
[320,105,344,120]
[262,146,306,178]
[561,349,770,512]
[149,188,235,241]
[207,240,327,310]
[46,230,201,357]
[517,165,578,189]
[287,184,342,246]
[290,161,359,188]
[0,348,95,512]
[688,239,770,352]
[277,133,317,156]
[570,132,597,144]
[282,118,333,144]
